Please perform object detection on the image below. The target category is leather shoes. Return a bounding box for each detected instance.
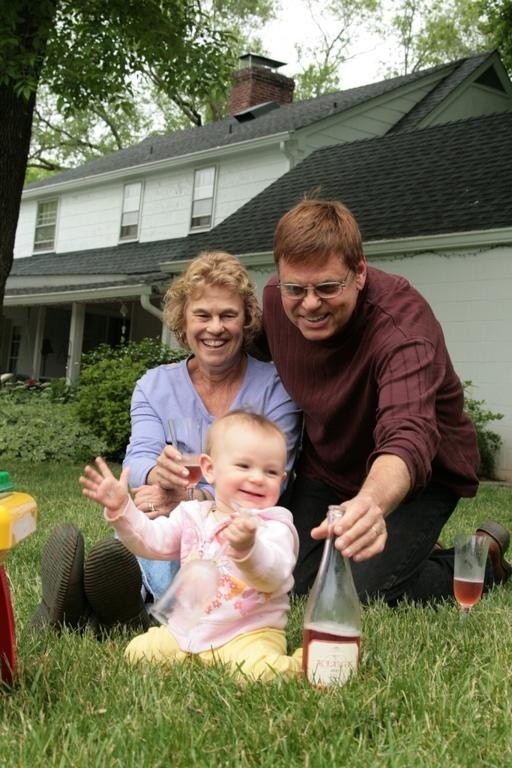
[474,521,512,587]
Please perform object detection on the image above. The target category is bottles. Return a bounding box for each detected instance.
[301,498,366,692]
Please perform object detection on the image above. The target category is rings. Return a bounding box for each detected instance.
[372,527,381,536]
[148,502,154,512]
[263,200,510,609]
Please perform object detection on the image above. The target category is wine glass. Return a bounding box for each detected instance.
[447,528,495,617]
[169,412,205,501]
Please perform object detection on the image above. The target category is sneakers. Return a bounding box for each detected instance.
[28,523,90,636]
[83,539,150,637]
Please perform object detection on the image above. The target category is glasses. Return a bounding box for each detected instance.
[275,264,352,300]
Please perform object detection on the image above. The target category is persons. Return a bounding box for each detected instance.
[26,250,301,634]
[78,412,303,690]
